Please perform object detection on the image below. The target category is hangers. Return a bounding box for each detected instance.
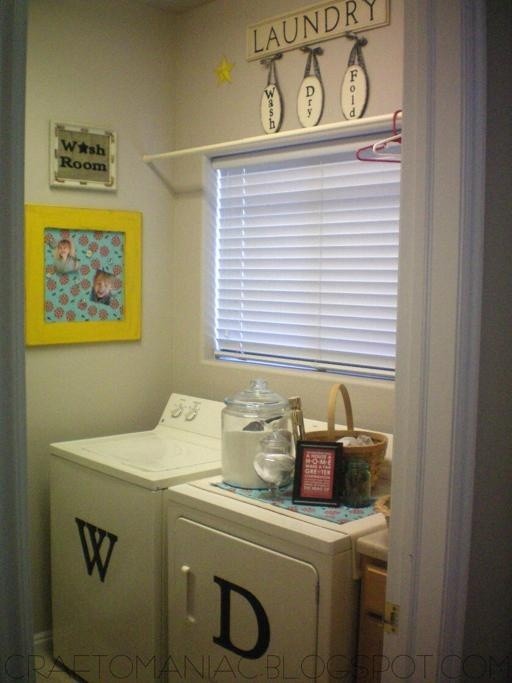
[373,133,401,157]
[357,110,405,163]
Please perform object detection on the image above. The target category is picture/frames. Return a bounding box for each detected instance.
[25,204,141,349]
[48,119,116,194]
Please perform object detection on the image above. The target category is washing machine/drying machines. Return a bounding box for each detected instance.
[166,415,394,677]
[48,390,295,678]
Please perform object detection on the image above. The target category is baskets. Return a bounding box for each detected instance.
[302,383,389,488]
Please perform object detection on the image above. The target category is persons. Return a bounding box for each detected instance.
[90,270,115,305]
[47,239,81,275]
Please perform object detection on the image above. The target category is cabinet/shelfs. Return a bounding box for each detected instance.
[356,559,386,683]
[160,473,382,683]
